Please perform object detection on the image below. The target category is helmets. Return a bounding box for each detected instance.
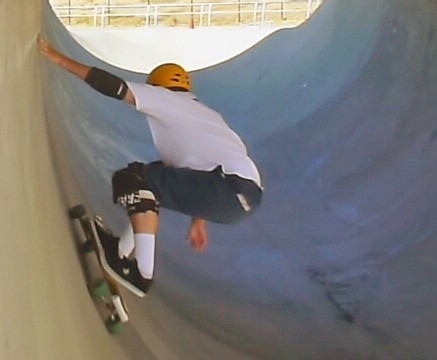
[146,63,192,91]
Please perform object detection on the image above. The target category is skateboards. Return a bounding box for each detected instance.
[69,203,131,330]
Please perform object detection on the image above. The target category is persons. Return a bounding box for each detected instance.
[36,30,263,298]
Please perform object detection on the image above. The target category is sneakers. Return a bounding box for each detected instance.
[98,250,152,299]
[89,219,120,254]
